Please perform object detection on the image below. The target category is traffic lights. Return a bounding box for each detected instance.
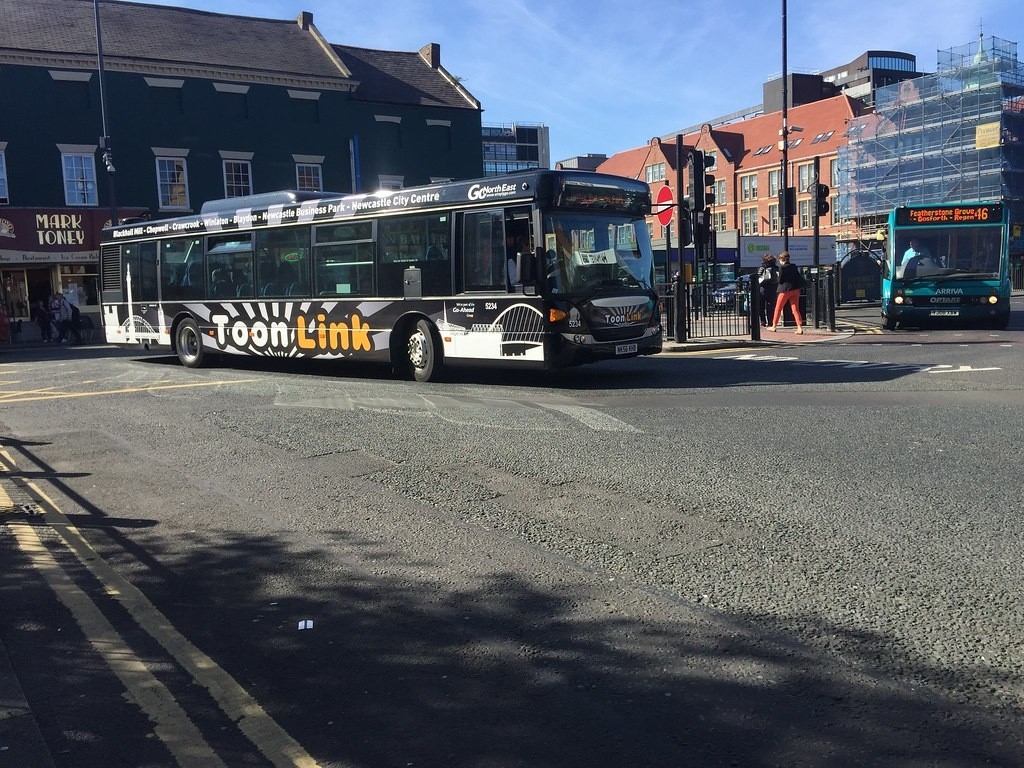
[817,181,829,215]
[705,157,716,206]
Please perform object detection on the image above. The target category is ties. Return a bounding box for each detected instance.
[915,250,918,258]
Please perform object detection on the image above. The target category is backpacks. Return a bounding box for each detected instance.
[63,300,80,320]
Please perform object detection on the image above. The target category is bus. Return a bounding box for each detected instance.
[880,198,1014,330]
[96,168,664,382]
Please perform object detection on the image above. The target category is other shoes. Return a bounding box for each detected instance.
[49,339,54,342]
[53,340,60,343]
[767,327,776,332]
[43,339,47,342]
[795,330,803,334]
[760,324,765,328]
[72,340,82,345]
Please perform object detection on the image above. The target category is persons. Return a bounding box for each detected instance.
[54,293,86,345]
[900,238,932,271]
[505,234,535,287]
[768,250,803,334]
[760,256,778,326]
[37,299,53,342]
[453,233,515,289]
[670,271,686,293]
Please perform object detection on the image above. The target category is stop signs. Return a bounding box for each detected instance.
[656,185,674,227]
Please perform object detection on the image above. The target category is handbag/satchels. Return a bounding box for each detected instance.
[758,265,772,284]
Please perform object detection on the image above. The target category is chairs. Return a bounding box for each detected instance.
[425,246,444,262]
[533,245,547,284]
[183,259,360,296]
[903,255,935,279]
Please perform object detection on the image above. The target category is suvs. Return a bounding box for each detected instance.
[713,273,751,313]
